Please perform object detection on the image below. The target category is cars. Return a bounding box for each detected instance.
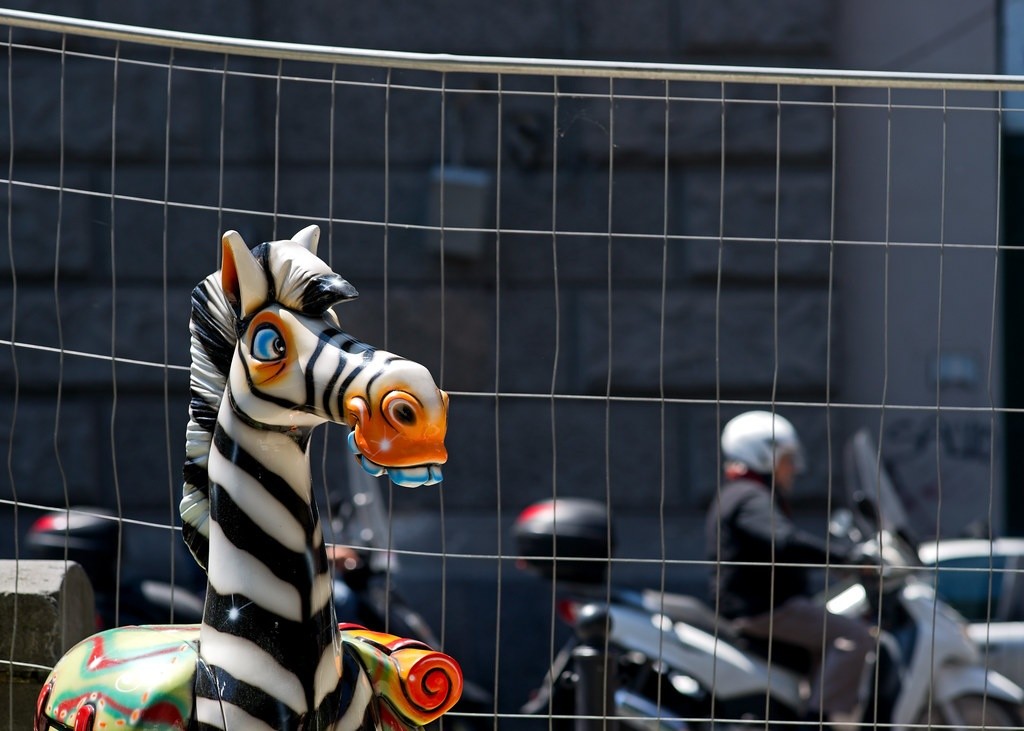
[818,535,1024,688]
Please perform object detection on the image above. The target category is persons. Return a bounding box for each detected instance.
[325,546,366,626]
[705,410,879,731]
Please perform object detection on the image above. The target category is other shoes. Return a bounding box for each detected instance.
[797,709,833,731]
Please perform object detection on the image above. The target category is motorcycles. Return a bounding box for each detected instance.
[514,427,1024,726]
[19,442,501,731]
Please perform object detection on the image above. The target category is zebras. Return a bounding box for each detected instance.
[177,225,451,731]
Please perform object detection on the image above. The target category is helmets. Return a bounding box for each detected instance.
[720,410,806,474]
